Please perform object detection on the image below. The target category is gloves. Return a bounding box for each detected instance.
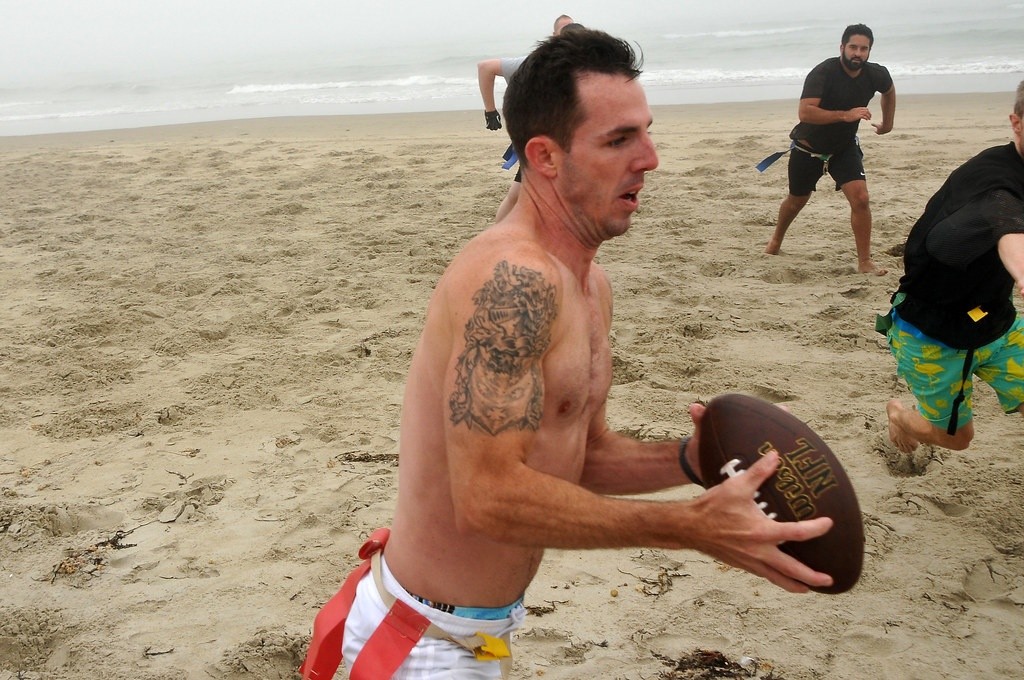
[485,109,502,131]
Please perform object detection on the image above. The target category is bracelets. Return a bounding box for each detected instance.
[678,436,704,488]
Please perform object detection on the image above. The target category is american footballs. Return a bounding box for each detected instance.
[696,394,864,595]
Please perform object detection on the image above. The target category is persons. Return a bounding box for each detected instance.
[876,79,1024,452]
[342,29,832,680]
[478,14,587,226]
[764,24,896,277]
